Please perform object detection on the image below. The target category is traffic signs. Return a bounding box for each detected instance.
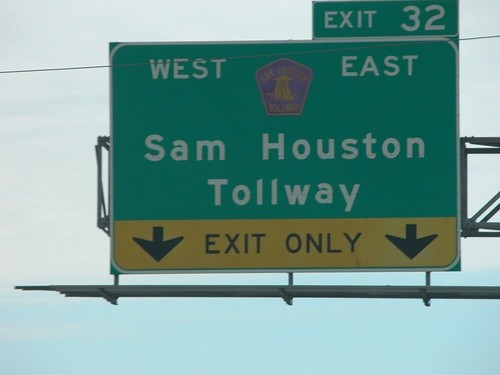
[109,37,461,274]
